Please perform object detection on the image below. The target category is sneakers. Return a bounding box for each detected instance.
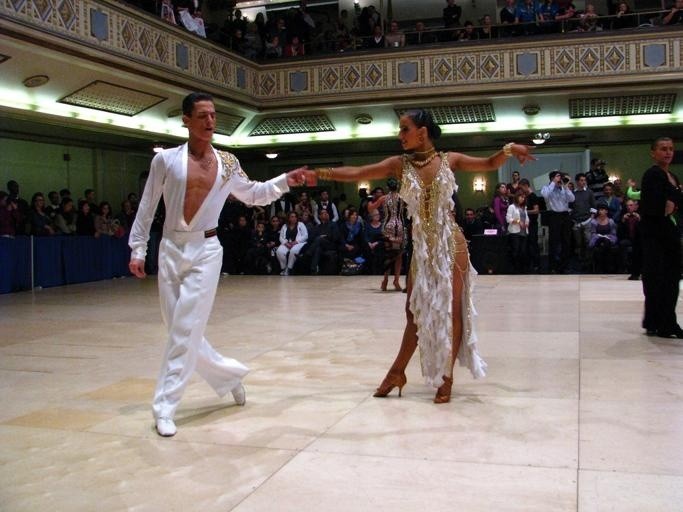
[641,322,682,339]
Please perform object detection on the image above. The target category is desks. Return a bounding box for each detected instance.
[471,234,504,274]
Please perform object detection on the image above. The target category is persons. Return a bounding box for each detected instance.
[367,178,408,290]
[452,161,680,281]
[296,108,540,405]
[216,188,413,276]
[2,180,166,239]
[639,136,683,340]
[119,0,682,61]
[127,91,307,436]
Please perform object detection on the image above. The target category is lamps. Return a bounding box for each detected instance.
[532,132,550,145]
[152,144,164,153]
[353,0,361,12]
[265,153,278,159]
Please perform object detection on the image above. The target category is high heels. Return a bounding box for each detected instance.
[432,373,453,405]
[378,278,388,292]
[391,280,402,291]
[371,372,408,399]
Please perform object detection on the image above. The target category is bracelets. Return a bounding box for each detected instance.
[316,167,334,181]
[502,142,515,158]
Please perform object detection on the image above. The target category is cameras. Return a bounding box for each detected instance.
[601,160,609,165]
[627,214,635,218]
[558,173,569,183]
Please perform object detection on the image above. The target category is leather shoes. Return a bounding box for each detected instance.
[155,416,177,437]
[230,382,246,406]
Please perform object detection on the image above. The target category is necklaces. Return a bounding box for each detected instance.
[187,143,214,171]
[414,147,435,155]
[405,152,439,167]
